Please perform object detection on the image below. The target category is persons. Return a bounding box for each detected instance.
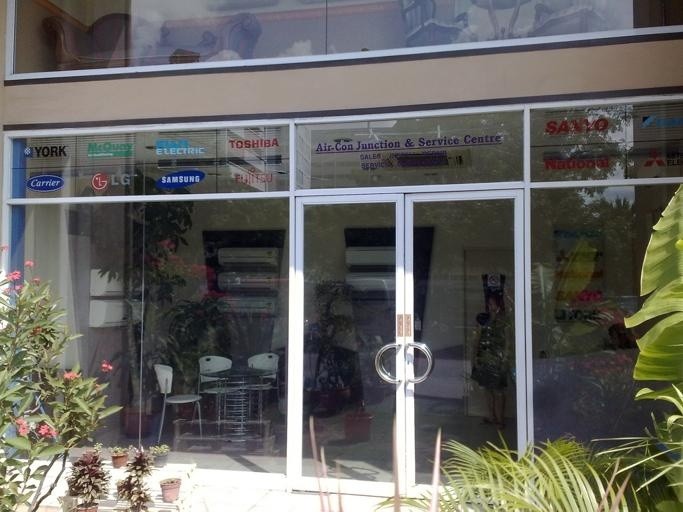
[469,292,513,431]
[324,282,375,420]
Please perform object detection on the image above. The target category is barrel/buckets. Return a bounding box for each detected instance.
[344,400,373,443]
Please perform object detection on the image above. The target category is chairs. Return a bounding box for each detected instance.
[152,352,281,447]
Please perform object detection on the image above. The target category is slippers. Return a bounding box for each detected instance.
[496,422,505,428]
[480,417,496,425]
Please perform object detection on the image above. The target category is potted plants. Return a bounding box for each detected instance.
[64,443,179,512]
